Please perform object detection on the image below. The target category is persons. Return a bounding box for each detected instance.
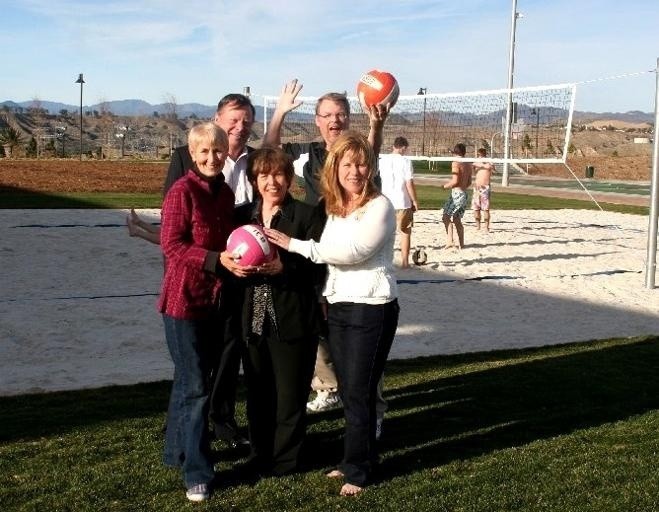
[262,78,391,439]
[236,148,326,484]
[471,148,495,233]
[262,130,400,498]
[163,93,261,441]
[157,122,258,503]
[440,143,472,252]
[376,135,418,272]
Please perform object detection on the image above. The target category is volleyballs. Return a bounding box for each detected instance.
[357,69,398,114]
[225,223,275,274]
[412,250,428,265]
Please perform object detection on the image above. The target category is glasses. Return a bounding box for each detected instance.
[317,112,348,120]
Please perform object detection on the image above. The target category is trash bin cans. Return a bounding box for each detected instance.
[585,165,594,178]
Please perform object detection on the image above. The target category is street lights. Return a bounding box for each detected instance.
[62,126,66,157]
[74,72,86,161]
[241,85,251,100]
[121,126,129,160]
[510,11,526,95]
[417,88,429,157]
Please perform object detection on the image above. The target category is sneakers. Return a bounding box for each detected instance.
[376,418,383,439]
[234,454,261,473]
[186,484,210,502]
[306,390,339,411]
